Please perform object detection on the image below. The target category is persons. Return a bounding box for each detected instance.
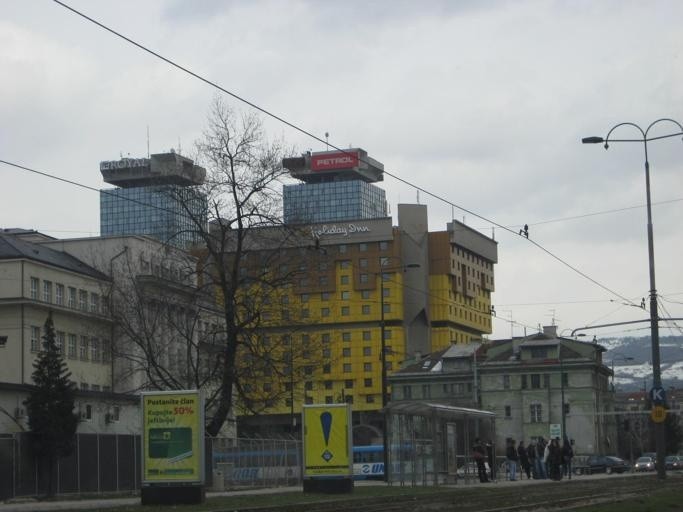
[473,435,576,483]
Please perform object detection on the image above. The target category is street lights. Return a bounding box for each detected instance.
[381,256,421,486]
[612,357,634,393]
[582,136,665,481]
[558,329,585,475]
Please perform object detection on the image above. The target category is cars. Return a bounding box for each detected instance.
[457,462,491,478]
[560,456,630,475]
[635,452,683,471]
